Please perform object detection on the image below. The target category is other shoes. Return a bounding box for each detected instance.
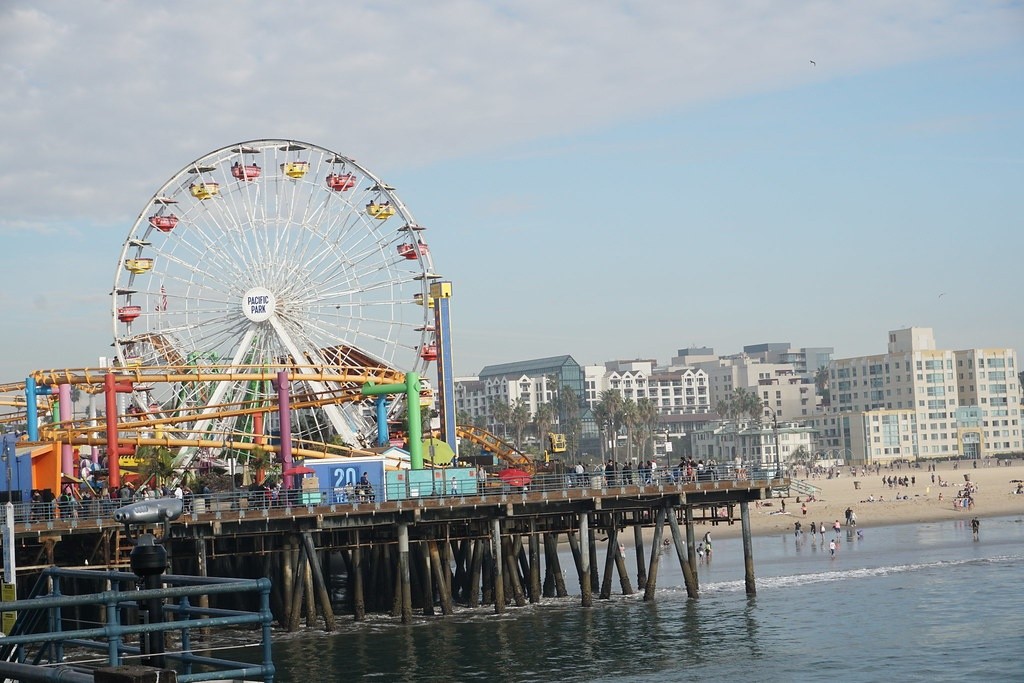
[851,525,853,527]
[855,525,856,526]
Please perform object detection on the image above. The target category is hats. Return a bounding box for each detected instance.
[609,459,612,462]
[453,476,455,478]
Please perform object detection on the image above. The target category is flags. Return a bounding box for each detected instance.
[155,286,167,312]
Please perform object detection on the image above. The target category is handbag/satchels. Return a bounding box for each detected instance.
[687,464,692,475]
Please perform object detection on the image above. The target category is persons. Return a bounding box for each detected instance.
[620,544,625,559]
[868,494,884,502]
[861,460,911,476]
[954,481,978,511]
[31,480,210,521]
[836,469,840,477]
[793,465,836,479]
[478,466,485,494]
[997,458,1012,467]
[1016,481,1023,494]
[720,509,727,517]
[451,476,457,495]
[882,475,915,487]
[896,492,901,500]
[973,461,977,468]
[664,538,670,546]
[569,455,747,488]
[346,472,375,503]
[971,517,979,540]
[755,495,856,540]
[697,532,711,560]
[256,479,284,506]
[829,539,835,555]
[851,467,856,477]
[929,459,958,500]
